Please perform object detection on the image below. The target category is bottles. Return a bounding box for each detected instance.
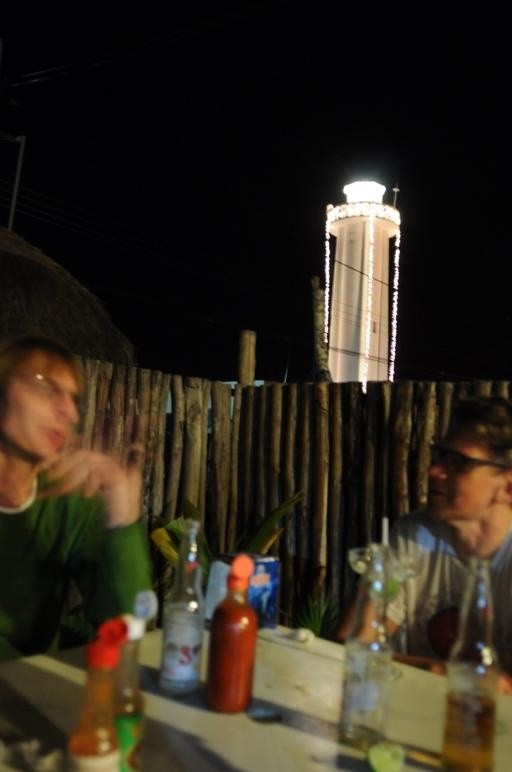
[337,544,394,751]
[159,518,207,693]
[65,588,159,772]
[440,556,498,770]
[205,554,259,715]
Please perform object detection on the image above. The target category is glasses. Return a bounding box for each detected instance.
[429,443,508,477]
[7,370,83,408]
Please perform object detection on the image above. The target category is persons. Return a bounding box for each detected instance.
[0,333,158,662]
[317,396,512,694]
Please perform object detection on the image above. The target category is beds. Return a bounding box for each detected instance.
[0,615,510,772]
